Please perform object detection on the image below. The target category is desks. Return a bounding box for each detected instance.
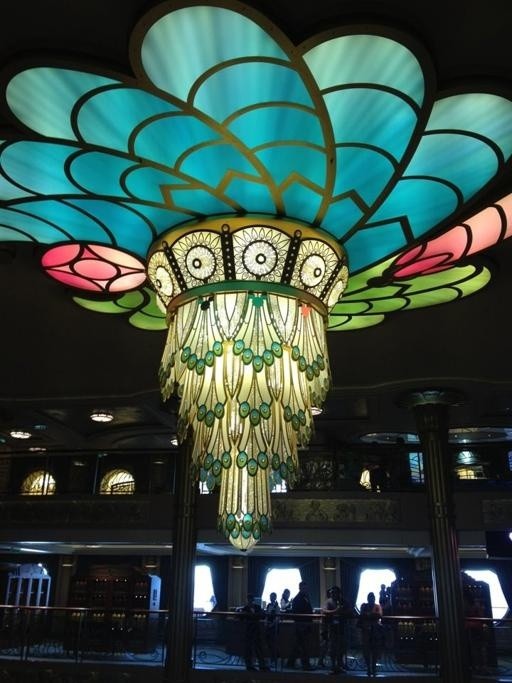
[224,617,321,659]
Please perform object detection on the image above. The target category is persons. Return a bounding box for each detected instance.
[285,580,318,671]
[379,583,392,616]
[240,591,270,672]
[321,583,346,672]
[278,587,292,610]
[356,590,383,677]
[265,589,282,630]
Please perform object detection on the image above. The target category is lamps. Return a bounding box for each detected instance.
[0,0,510,553]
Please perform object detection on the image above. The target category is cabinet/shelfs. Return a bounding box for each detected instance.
[61,572,161,654]
[388,561,498,668]
[0,563,52,649]
